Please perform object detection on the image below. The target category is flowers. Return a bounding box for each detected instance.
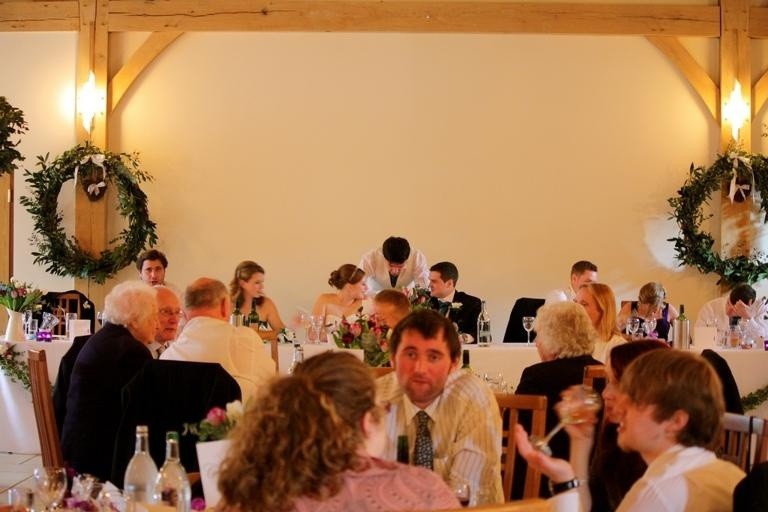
[0,341,54,402]
[331,306,392,367]
[450,302,462,311]
[404,284,434,312]
[83,300,91,309]
[0,277,49,314]
[190,400,245,441]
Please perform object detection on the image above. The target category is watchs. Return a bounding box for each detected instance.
[548,476,580,496]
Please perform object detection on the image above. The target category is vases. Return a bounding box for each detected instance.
[5,308,26,342]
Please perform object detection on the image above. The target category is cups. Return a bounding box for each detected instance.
[40,313,60,331]
[66,313,77,338]
[8,488,32,512]
[727,325,740,350]
[28,319,37,337]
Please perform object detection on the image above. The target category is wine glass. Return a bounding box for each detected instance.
[646,318,657,332]
[627,319,639,337]
[641,322,653,337]
[300,313,312,346]
[530,388,602,457]
[97,312,102,326]
[472,367,510,392]
[705,318,718,345]
[311,316,324,346]
[22,310,33,336]
[445,480,470,508]
[522,317,534,347]
[737,319,750,349]
[33,467,66,512]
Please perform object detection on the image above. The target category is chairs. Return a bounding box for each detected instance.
[582,365,607,470]
[27,349,63,469]
[495,393,548,497]
[433,497,558,512]
[502,297,546,344]
[52,294,80,334]
[720,411,768,474]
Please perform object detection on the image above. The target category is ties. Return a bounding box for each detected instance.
[413,410,433,470]
[158,345,165,357]
[731,315,740,327]
[438,301,450,318]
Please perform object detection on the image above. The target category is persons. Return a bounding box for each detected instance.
[216,350,463,512]
[229,259,285,339]
[370,308,505,509]
[292,235,493,346]
[554,339,673,512]
[616,281,679,342]
[543,260,598,304]
[60,280,204,501]
[572,281,629,363]
[696,284,768,347]
[136,249,187,334]
[144,285,184,359]
[501,301,604,501]
[513,347,747,512]
[158,277,276,409]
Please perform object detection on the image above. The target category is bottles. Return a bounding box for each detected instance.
[290,348,304,374]
[287,344,301,375]
[248,299,259,331]
[460,350,473,372]
[229,298,245,326]
[123,426,158,512]
[672,304,690,350]
[151,432,192,512]
[476,300,491,347]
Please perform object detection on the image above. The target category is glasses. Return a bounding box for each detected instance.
[159,309,186,316]
